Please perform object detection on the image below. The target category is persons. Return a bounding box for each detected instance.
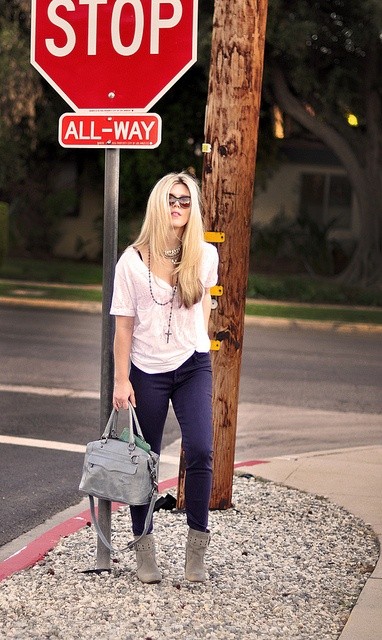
[108,170,221,587]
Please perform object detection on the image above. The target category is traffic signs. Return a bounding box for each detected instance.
[58,114,162,148]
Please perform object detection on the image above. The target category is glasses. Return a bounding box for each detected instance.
[165,193,190,208]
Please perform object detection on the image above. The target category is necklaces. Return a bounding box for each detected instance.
[154,245,182,264]
[145,240,186,343]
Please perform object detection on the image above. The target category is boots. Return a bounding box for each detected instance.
[131,532,163,584]
[184,527,212,585]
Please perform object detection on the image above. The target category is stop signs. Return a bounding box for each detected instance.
[30,0,200,111]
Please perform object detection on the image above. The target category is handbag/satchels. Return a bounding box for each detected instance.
[77,397,160,508]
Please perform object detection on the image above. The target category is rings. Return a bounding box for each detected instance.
[117,403,123,408]
